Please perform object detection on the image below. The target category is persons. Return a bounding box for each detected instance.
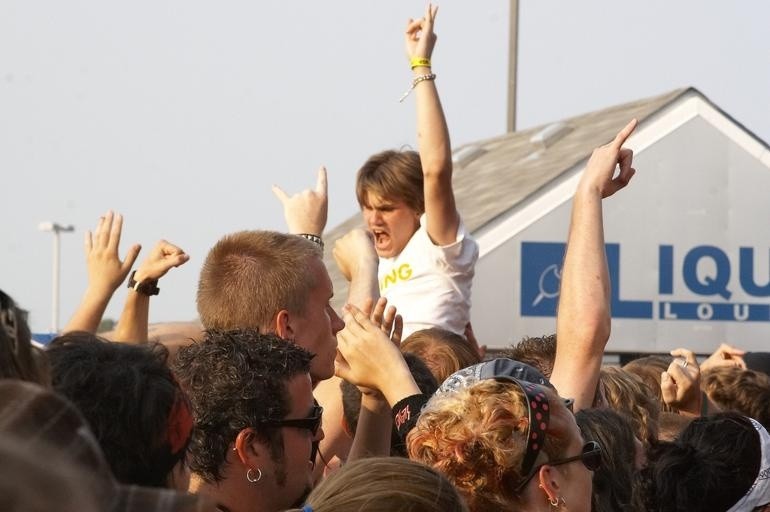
[633,412,770,511]
[354,0,486,361]
[340,381,361,437]
[302,459,464,512]
[700,343,748,372]
[311,226,381,484]
[574,409,658,512]
[46,332,194,487]
[169,328,324,512]
[406,375,592,512]
[196,229,344,394]
[346,297,402,463]
[62,210,141,332]
[334,305,431,459]
[661,348,703,417]
[404,328,477,387]
[96,237,189,345]
[271,165,326,257]
[551,120,638,412]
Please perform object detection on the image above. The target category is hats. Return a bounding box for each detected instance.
[0,378,199,512]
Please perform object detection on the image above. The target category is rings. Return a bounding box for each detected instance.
[681,361,689,369]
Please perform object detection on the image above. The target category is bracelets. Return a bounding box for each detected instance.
[296,233,323,250]
[410,57,432,69]
[399,74,437,102]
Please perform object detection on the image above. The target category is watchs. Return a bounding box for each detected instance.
[127,270,159,296]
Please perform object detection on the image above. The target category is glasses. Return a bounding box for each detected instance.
[513,441,605,497]
[562,397,576,417]
[247,397,324,436]
